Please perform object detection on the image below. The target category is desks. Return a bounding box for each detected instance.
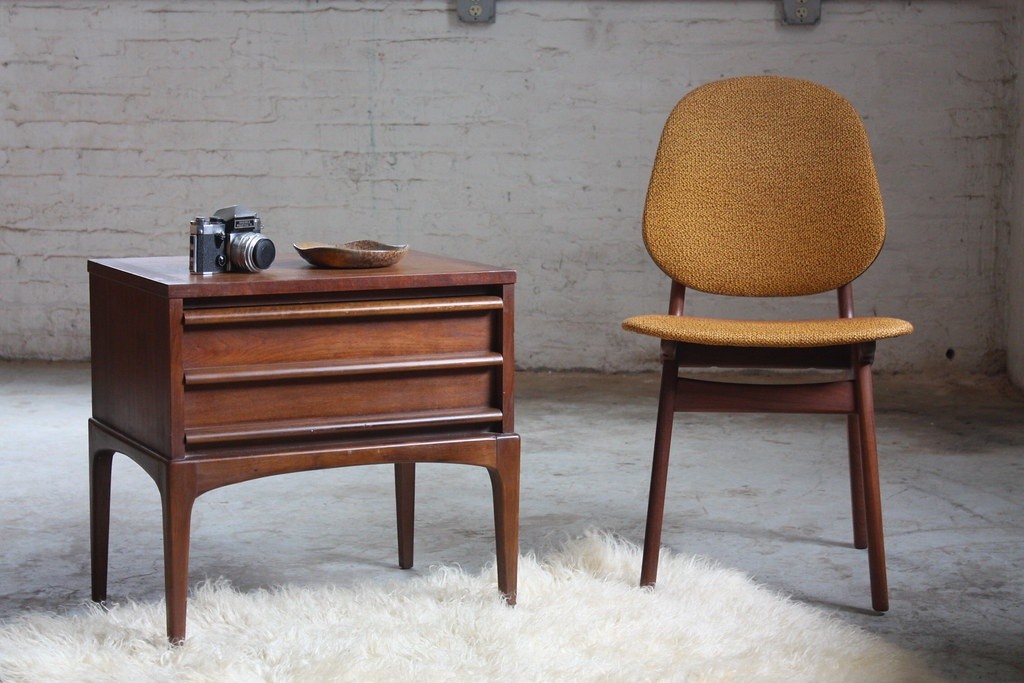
[85,240,526,647]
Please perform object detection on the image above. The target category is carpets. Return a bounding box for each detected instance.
[0,527,950,683]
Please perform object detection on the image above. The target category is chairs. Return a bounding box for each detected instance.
[621,73,916,615]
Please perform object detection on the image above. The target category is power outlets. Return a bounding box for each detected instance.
[783,0,821,25]
[456,0,495,23]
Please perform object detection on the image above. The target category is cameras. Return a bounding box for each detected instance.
[188,204,276,277]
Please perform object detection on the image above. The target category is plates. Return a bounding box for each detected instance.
[293,240,409,268]
